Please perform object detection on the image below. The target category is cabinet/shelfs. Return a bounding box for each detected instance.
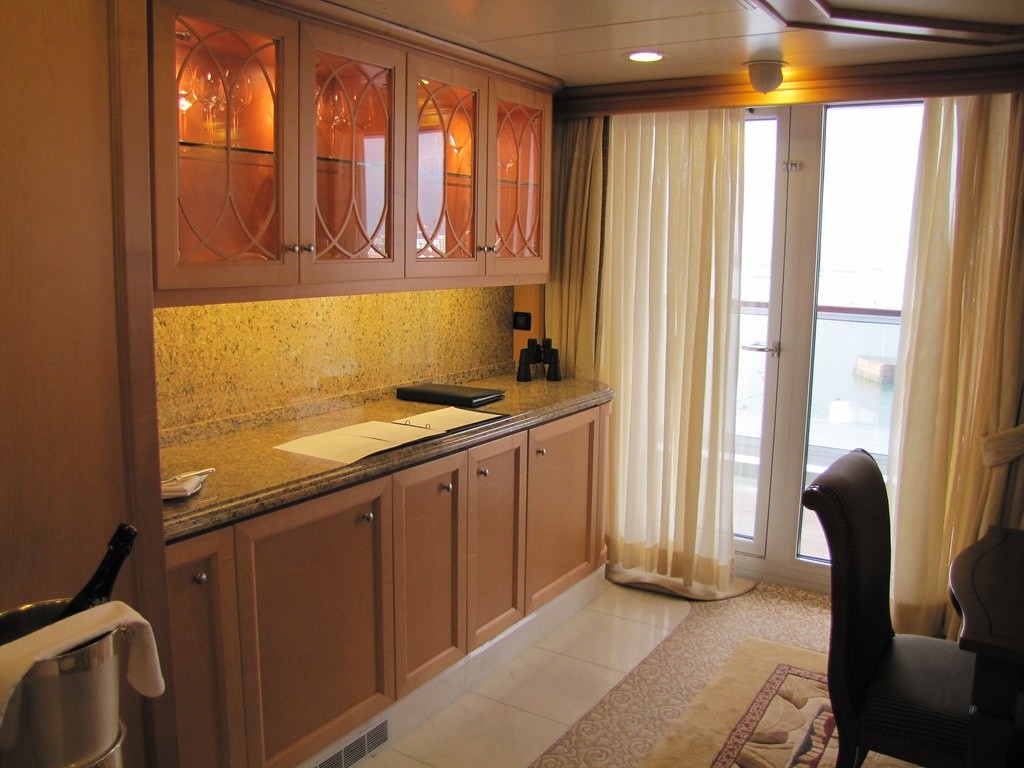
[165,403,612,768]
[145,0,553,289]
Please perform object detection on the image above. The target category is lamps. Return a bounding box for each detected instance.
[749,63,784,93]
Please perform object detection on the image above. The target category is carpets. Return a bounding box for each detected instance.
[530,583,924,768]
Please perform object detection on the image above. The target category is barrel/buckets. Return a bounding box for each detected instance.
[0,598,129,768]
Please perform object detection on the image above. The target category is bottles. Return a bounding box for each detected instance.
[52,520,140,656]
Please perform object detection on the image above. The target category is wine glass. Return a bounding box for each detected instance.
[448,118,472,175]
[174,56,253,150]
[314,84,376,163]
[496,139,518,181]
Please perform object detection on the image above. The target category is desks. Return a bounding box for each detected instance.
[950,526,1024,768]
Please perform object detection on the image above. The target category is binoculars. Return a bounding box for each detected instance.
[517,338,561,382]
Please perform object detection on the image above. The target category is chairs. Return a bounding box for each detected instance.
[802,448,976,768]
